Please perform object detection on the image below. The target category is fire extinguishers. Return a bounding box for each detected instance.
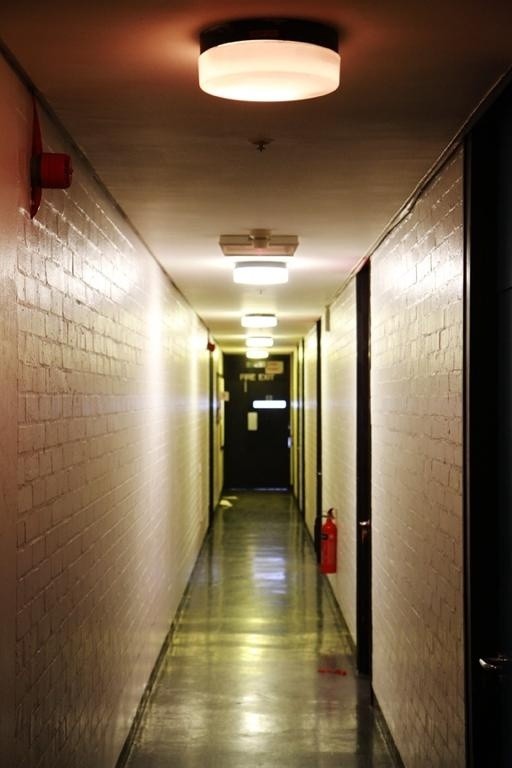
[321,508,336,574]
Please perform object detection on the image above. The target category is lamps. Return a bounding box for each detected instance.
[233,260,290,286]
[197,18,343,105]
